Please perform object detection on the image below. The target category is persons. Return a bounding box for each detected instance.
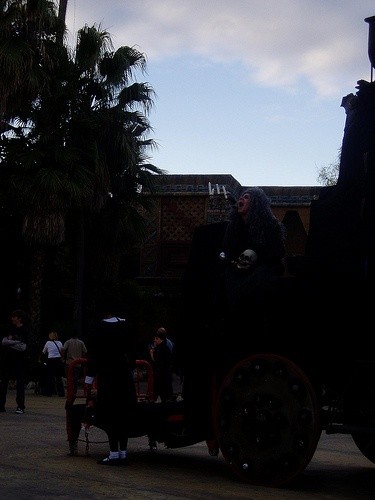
[147,326,175,354]
[0,309,28,414]
[92,333,138,466]
[186,187,286,372]
[39,331,65,397]
[147,331,174,404]
[60,327,87,397]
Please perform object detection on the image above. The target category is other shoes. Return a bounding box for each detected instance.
[16,406,25,414]
[97,456,118,465]
[119,457,128,465]
